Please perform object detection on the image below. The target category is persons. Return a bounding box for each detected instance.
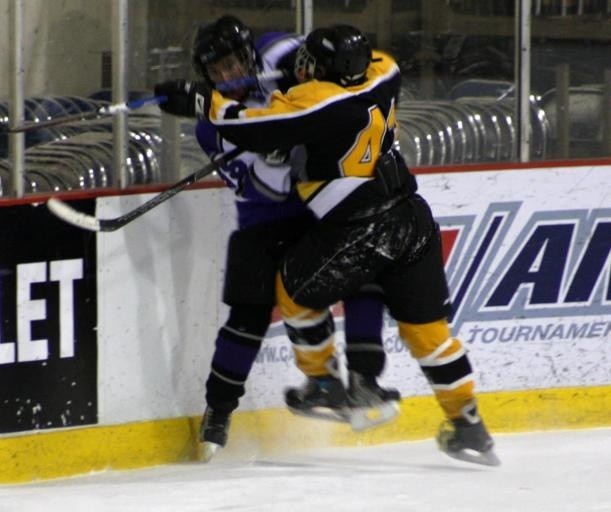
[154,24,494,452]
[192,16,401,448]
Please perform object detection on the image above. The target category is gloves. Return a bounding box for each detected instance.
[154,79,213,123]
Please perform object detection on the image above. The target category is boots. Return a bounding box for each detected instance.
[286,356,346,410]
[437,397,493,453]
[198,405,230,448]
[344,365,400,408]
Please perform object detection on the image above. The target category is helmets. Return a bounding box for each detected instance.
[189,14,264,102]
[293,25,371,86]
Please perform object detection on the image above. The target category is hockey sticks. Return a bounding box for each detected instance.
[47,148,247,232]
[5,70,287,133]
